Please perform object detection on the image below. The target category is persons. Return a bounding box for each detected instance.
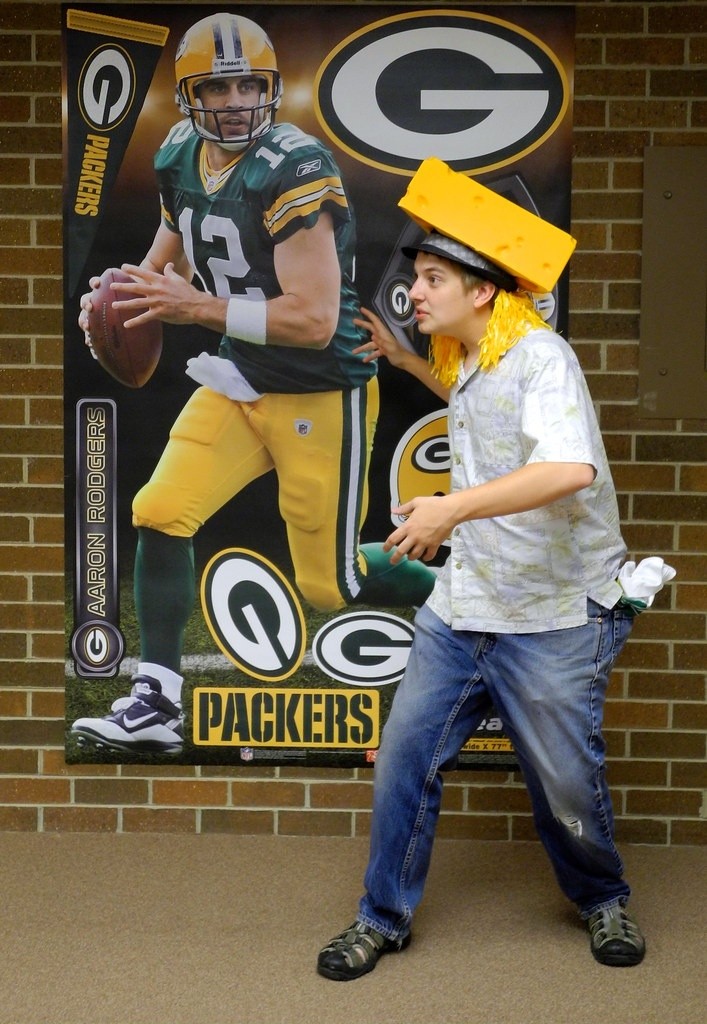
[70,15,437,761]
[317,234,647,981]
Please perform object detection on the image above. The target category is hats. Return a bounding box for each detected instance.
[396,155,578,294]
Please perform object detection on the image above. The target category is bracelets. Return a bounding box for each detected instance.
[224,297,268,348]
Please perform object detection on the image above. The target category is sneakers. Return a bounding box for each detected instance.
[316,920,413,981]
[70,674,185,757]
[585,901,647,968]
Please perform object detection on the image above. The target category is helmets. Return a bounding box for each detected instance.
[174,11,284,153]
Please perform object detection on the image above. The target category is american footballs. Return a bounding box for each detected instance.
[86,266,165,390]
